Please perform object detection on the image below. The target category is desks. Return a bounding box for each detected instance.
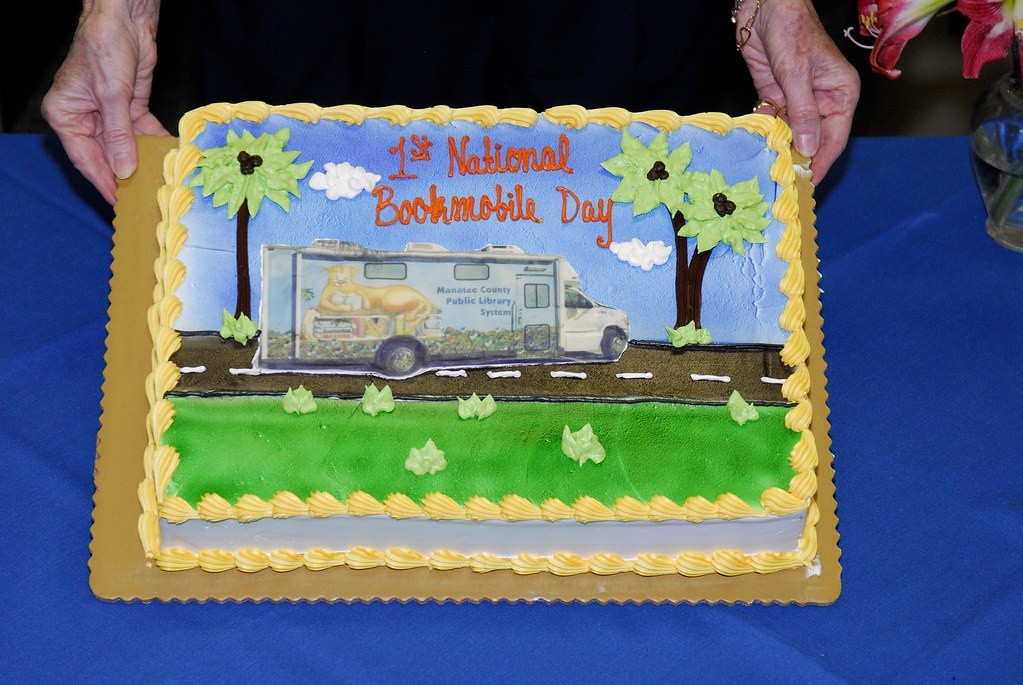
[1,131,1023,683]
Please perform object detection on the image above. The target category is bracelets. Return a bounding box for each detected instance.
[727,0,762,52]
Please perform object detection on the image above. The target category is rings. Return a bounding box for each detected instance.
[779,111,789,117]
[753,99,777,113]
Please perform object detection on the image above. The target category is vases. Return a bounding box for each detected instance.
[971,35,1023,253]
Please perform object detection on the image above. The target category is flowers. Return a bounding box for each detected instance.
[844,0,1023,114]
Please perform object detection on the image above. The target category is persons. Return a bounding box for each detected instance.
[40,0,861,211]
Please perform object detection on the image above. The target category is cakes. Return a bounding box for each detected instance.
[139,101,820,578]
